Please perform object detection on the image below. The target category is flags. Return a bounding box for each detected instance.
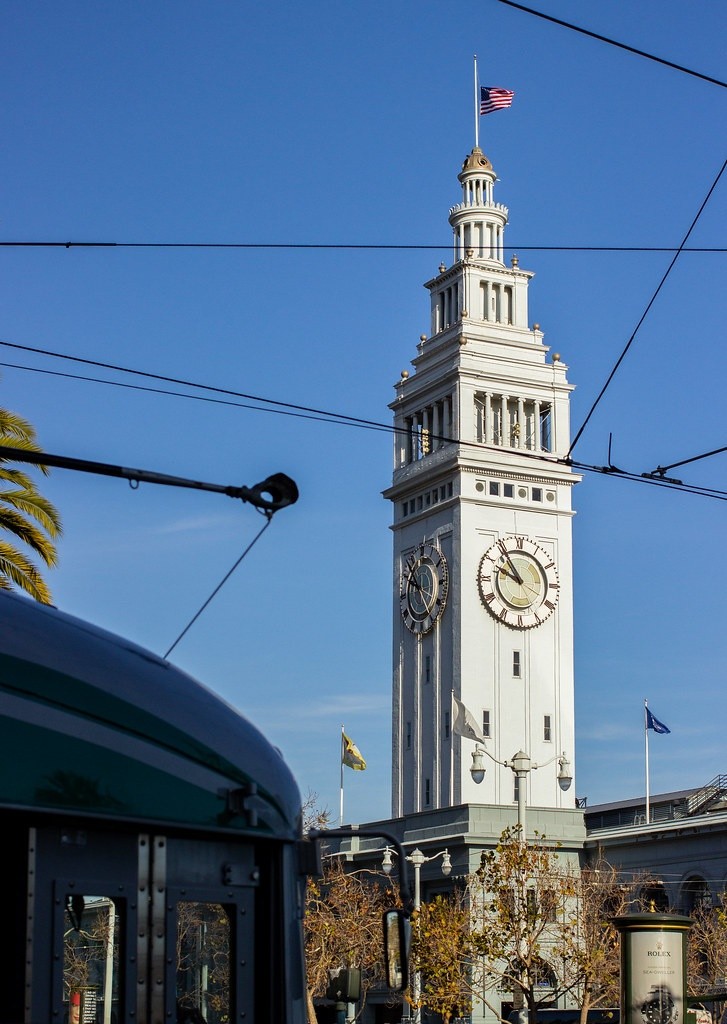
[450,696,485,745]
[480,86,514,115]
[644,706,671,734]
[341,731,366,771]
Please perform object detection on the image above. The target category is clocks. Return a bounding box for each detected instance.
[478,536,561,630]
[641,990,679,1024]
[398,544,449,635]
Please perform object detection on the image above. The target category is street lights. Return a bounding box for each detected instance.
[469,742,573,1009]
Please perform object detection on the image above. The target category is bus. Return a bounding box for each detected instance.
[0,444,414,1024]
